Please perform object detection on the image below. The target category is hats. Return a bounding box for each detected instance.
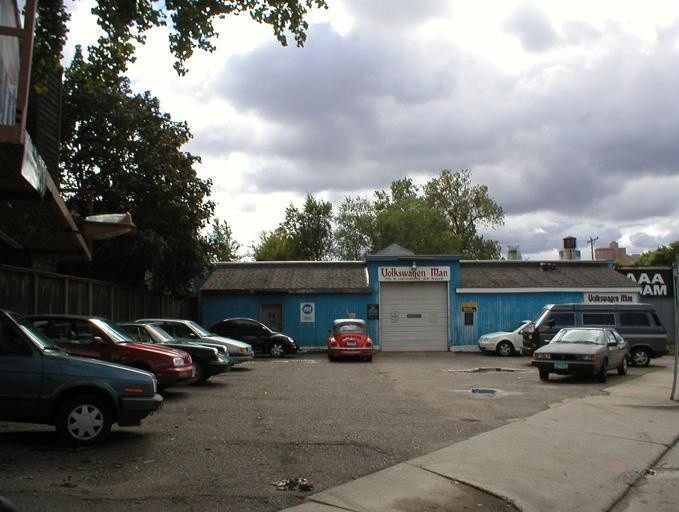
[479,318,533,357]
[327,318,376,362]
[531,328,630,382]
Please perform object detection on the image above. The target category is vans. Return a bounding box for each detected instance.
[523,301,669,367]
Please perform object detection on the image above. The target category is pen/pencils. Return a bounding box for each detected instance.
[523,301,669,367]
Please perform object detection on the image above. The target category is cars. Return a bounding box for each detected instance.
[327,318,376,362]
[207,318,298,359]
[134,318,255,368]
[2,310,164,443]
[531,328,630,382]
[20,313,196,394]
[479,318,533,357]
[113,322,234,387]
